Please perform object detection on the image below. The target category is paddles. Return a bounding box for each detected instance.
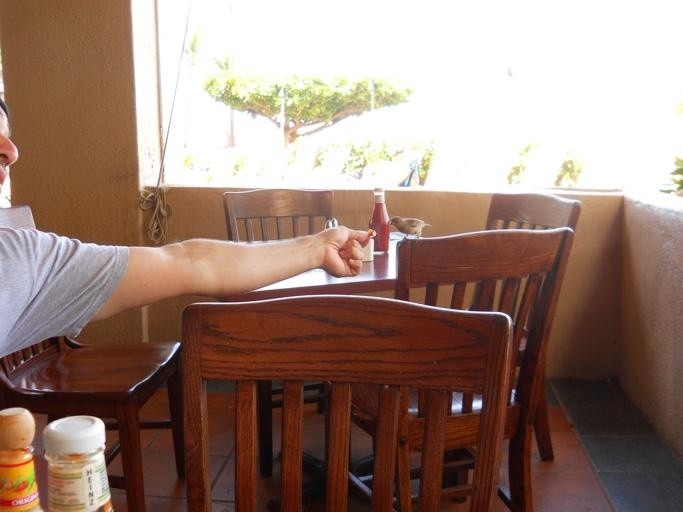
[492,398,613,512]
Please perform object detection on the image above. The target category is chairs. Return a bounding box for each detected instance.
[222,188,353,478]
[326,191,580,507]
[182,293,514,508]
[1,205,184,511]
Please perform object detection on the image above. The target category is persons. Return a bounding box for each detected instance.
[0,93,379,360]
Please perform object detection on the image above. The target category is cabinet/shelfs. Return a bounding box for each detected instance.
[43,415,115,512]
[368,187,390,252]
[0,406,44,512]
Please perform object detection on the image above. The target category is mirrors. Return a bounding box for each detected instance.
[389,216,433,239]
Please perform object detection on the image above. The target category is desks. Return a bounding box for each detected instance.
[215,234,427,470]
